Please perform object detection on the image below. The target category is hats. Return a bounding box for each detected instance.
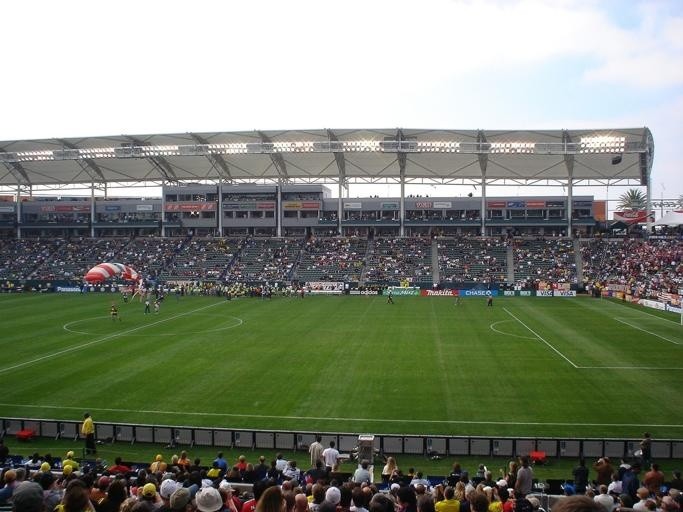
[142,479,235,512]
[325,486,341,505]
[156,453,162,462]
[4,450,74,482]
[98,475,112,487]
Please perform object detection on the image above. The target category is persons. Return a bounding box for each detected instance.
[1,436,683,512]
[143,299,150,313]
[80,412,97,455]
[640,432,651,466]
[110,298,122,321]
[0,194,682,308]
[152,299,160,313]
